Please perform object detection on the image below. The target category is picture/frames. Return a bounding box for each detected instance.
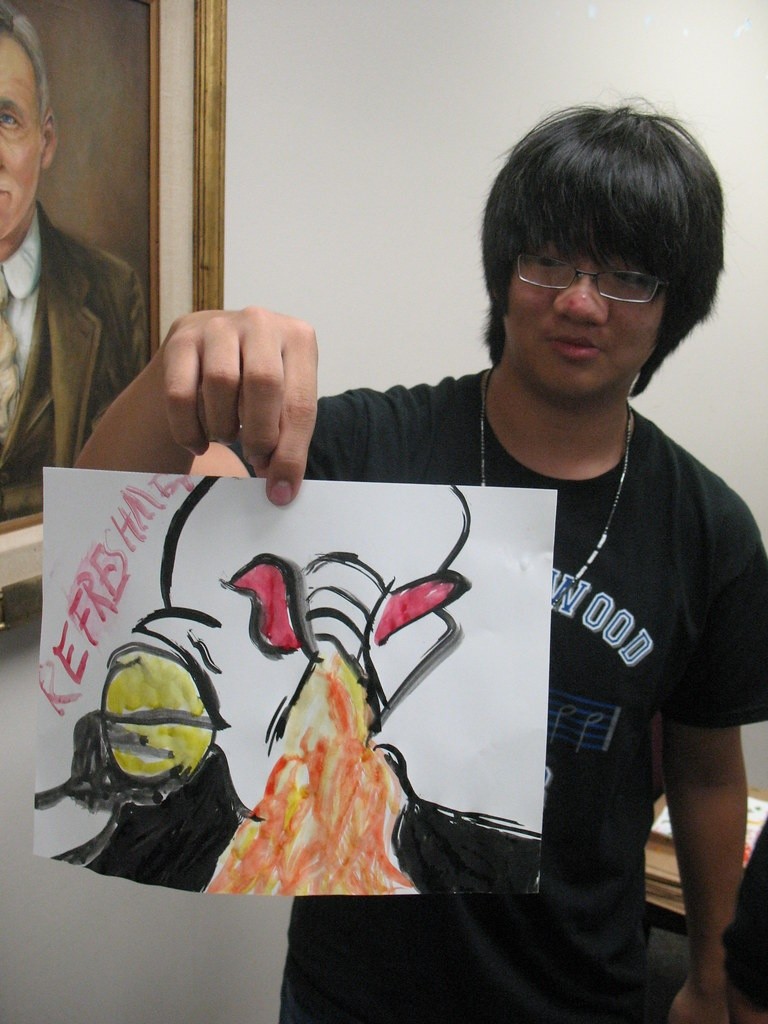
[0,1,227,585]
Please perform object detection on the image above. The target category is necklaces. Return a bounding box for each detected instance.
[480,366,631,614]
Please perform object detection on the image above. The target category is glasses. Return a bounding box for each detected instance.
[517,253,664,302]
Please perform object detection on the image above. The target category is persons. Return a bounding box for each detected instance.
[75,104,768,1024]
